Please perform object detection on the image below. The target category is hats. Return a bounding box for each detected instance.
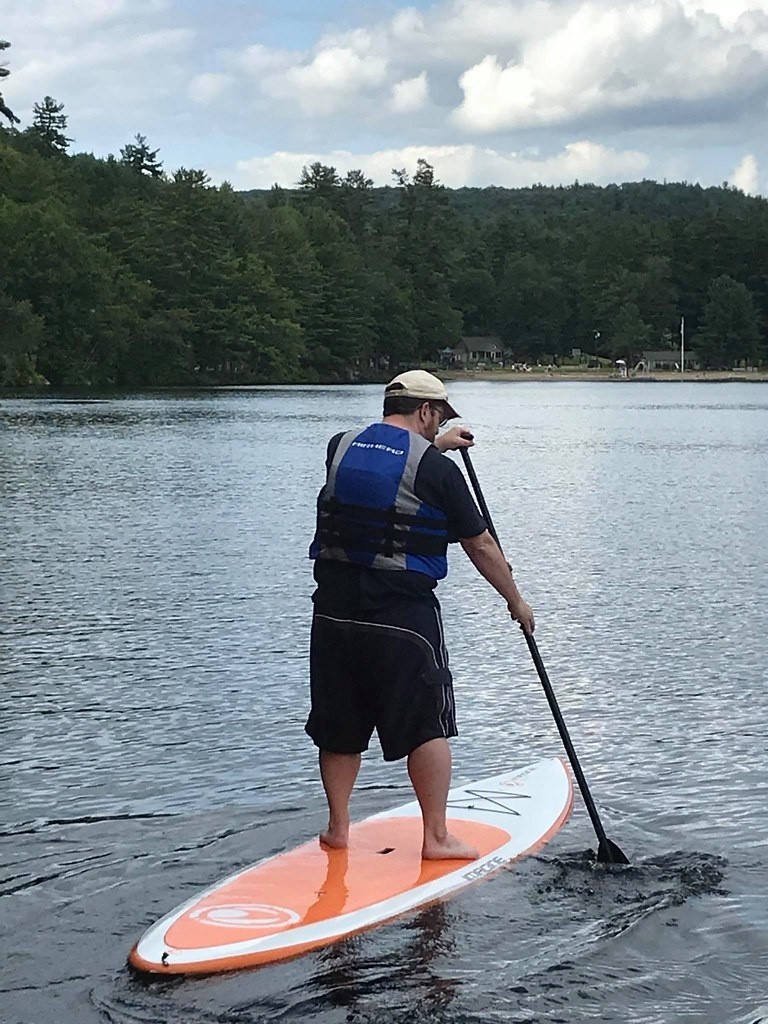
[384,369,462,420]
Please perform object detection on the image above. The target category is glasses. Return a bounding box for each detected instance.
[431,404,449,427]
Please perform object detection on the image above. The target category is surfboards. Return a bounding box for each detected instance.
[123,753,577,979]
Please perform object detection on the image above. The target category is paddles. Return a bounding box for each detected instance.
[451,429,628,872]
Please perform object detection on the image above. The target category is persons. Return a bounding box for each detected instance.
[305,371,535,859]
